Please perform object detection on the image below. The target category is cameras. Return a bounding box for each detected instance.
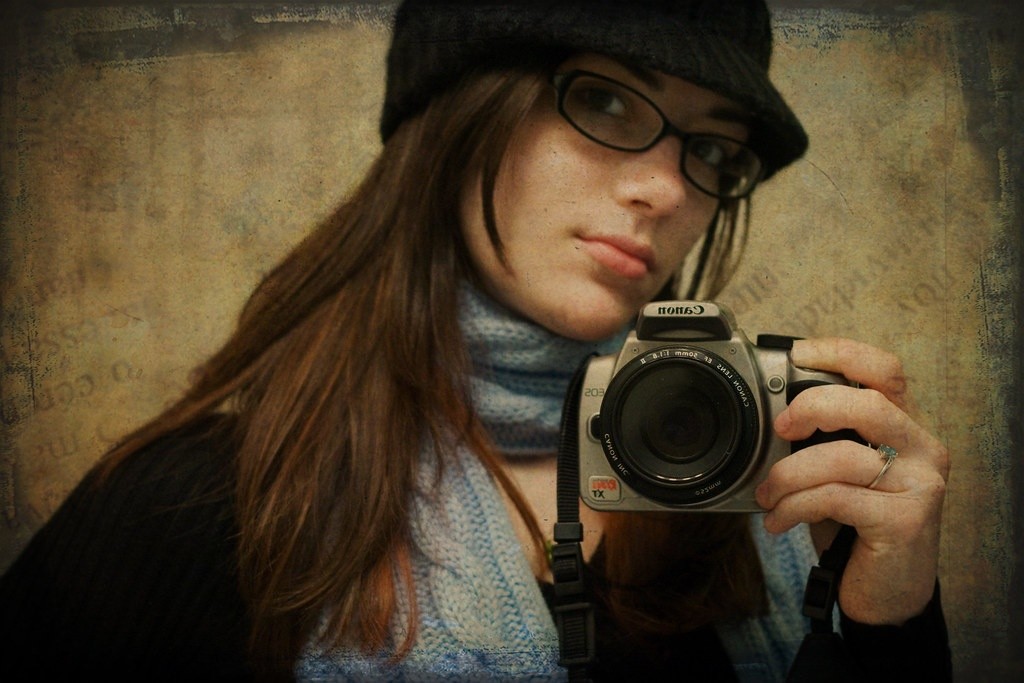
[578,300,865,518]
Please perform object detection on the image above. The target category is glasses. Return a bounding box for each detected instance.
[540,70,765,201]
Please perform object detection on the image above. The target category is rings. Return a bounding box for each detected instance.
[867,442,899,490]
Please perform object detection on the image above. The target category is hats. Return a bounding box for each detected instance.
[379,0,809,188]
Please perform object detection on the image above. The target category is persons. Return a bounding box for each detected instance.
[2,0,958,683]
